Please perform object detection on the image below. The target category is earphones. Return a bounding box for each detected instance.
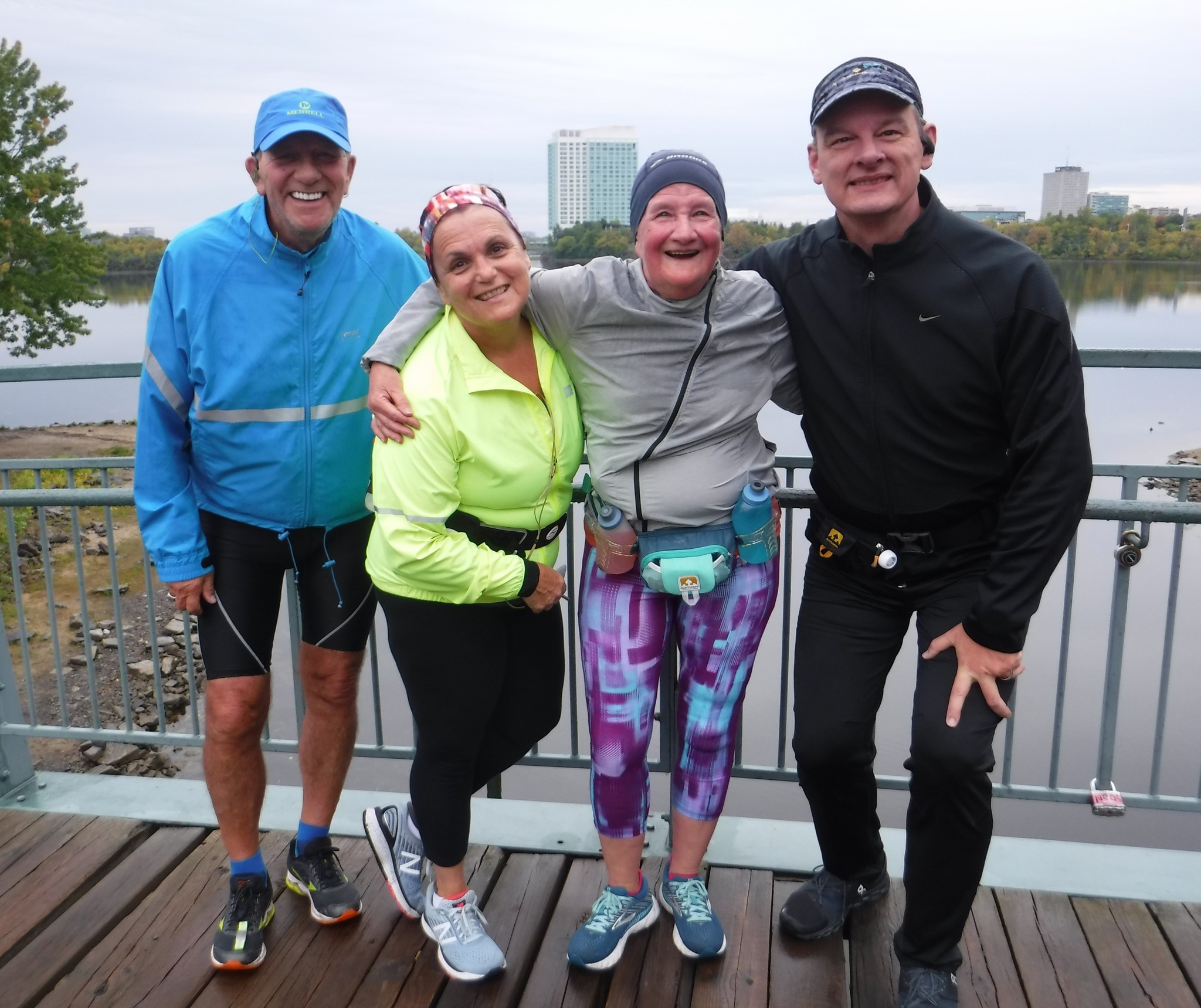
[253,159,259,182]
[920,130,934,156]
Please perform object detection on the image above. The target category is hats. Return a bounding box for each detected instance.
[810,56,924,126]
[253,86,352,156]
[629,148,728,244]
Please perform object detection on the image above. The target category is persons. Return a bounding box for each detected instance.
[129,90,439,970]
[360,178,592,988]
[730,51,1098,1008]
[361,145,803,972]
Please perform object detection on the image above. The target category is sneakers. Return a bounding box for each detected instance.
[660,852,728,963]
[566,868,660,974]
[420,880,507,984]
[210,869,276,971]
[286,838,362,925]
[779,849,891,942]
[362,800,429,919]
[898,965,959,1008]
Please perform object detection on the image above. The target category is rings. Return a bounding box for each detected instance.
[166,591,176,602]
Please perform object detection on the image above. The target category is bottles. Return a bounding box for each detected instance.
[595,504,637,574]
[732,479,780,563]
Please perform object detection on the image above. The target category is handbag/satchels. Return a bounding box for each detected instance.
[634,520,736,606]
[445,510,529,558]
[805,503,909,587]
